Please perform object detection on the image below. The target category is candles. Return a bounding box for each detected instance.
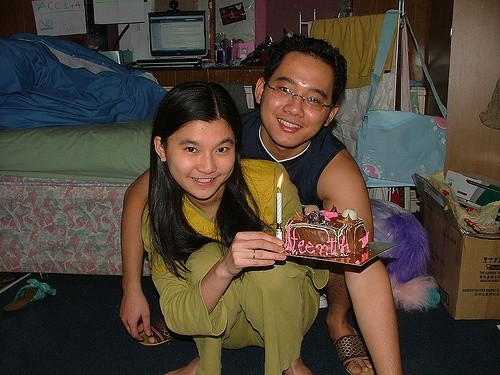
[276,173,283,240]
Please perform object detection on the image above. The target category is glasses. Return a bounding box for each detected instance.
[263,76,336,111]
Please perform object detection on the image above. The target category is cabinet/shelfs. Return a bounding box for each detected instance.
[146,68,264,86]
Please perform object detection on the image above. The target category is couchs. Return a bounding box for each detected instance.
[0,32,255,276]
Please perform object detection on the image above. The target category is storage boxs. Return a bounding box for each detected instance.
[411,170,500,319]
[100,51,133,66]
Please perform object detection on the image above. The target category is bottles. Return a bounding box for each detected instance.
[217,48,223,62]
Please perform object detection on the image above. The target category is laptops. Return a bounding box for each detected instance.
[132,11,210,69]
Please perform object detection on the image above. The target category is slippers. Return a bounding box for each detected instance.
[137,318,174,346]
[332,326,374,375]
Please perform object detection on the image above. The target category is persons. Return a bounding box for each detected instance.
[119,35,403,375]
[141,79,330,375]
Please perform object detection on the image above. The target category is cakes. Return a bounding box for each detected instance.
[282,204,369,258]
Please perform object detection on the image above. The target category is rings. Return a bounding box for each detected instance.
[253,250,255,258]
